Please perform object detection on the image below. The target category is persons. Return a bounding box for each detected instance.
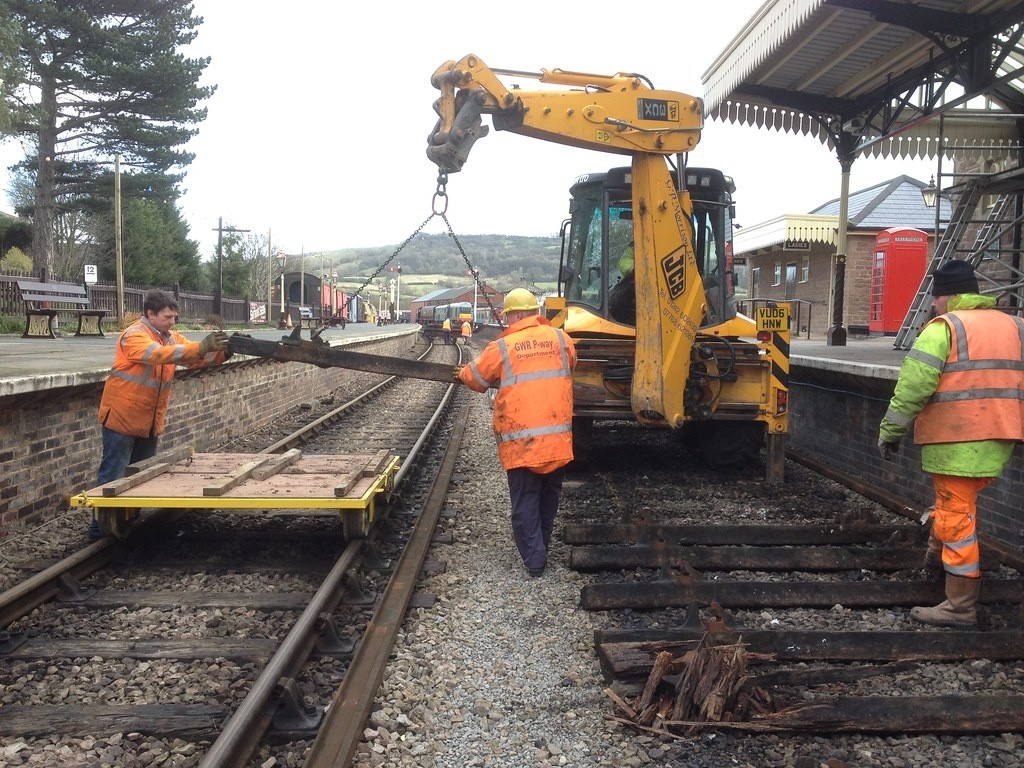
[442,318,454,344]
[443,287,578,579]
[459,320,472,346]
[87,287,235,540]
[874,259,1023,629]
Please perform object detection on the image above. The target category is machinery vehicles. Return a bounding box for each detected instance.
[421,54,773,475]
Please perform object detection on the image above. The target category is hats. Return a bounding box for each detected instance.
[931,259,981,296]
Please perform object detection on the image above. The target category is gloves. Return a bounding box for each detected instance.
[225,331,251,358]
[199,330,230,357]
[878,437,900,461]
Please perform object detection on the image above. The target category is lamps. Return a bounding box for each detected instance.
[922,174,953,210]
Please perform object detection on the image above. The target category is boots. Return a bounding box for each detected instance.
[909,517,982,626]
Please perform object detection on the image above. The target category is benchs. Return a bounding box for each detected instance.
[16,280,111,340]
[298,306,321,328]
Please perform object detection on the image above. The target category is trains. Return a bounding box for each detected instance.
[416,302,471,337]
[275,272,411,324]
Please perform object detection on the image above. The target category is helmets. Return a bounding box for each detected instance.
[499,287,540,317]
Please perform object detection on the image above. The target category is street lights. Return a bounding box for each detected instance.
[275,251,288,330]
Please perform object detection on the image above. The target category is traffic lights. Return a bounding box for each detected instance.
[475,271,479,276]
[398,268,402,273]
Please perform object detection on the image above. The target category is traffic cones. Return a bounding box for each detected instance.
[286,314,292,330]
